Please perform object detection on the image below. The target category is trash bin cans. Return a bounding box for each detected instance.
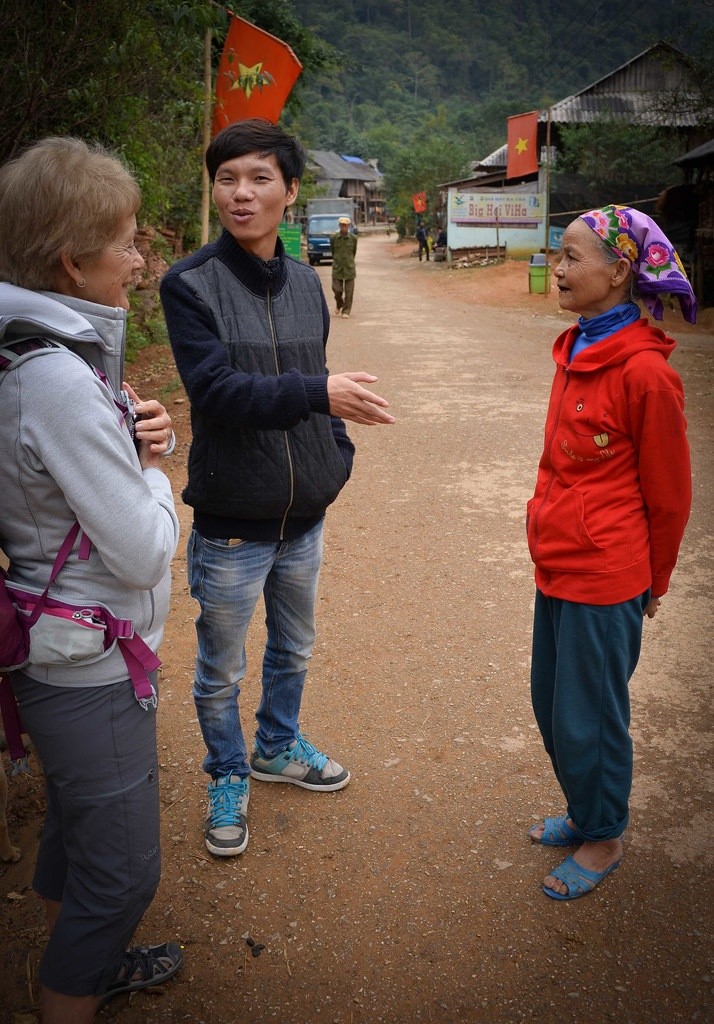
[528,253,551,295]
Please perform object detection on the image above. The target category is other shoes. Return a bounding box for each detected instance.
[342,312,349,319]
[335,306,340,314]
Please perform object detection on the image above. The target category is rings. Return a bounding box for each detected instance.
[164,430,168,440]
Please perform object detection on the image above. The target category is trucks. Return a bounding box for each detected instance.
[302,197,359,264]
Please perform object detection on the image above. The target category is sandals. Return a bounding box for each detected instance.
[66,938,184,1015]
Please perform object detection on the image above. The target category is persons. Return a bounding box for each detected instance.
[0,137,182,1024]
[525,202,696,903]
[330,217,359,319]
[159,117,396,855]
[432,226,447,253]
[416,220,431,262]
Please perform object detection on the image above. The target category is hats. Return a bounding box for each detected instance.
[338,217,351,224]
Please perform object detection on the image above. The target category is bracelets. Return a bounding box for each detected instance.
[168,438,172,449]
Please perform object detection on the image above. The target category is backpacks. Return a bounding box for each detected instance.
[1,336,138,672]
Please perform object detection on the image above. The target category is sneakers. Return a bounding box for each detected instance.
[205,770,250,856]
[248,733,351,792]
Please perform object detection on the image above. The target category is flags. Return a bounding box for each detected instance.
[506,111,539,179]
[413,192,427,213]
[211,14,302,138]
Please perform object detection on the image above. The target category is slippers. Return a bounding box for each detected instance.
[529,814,584,847]
[543,843,624,900]
